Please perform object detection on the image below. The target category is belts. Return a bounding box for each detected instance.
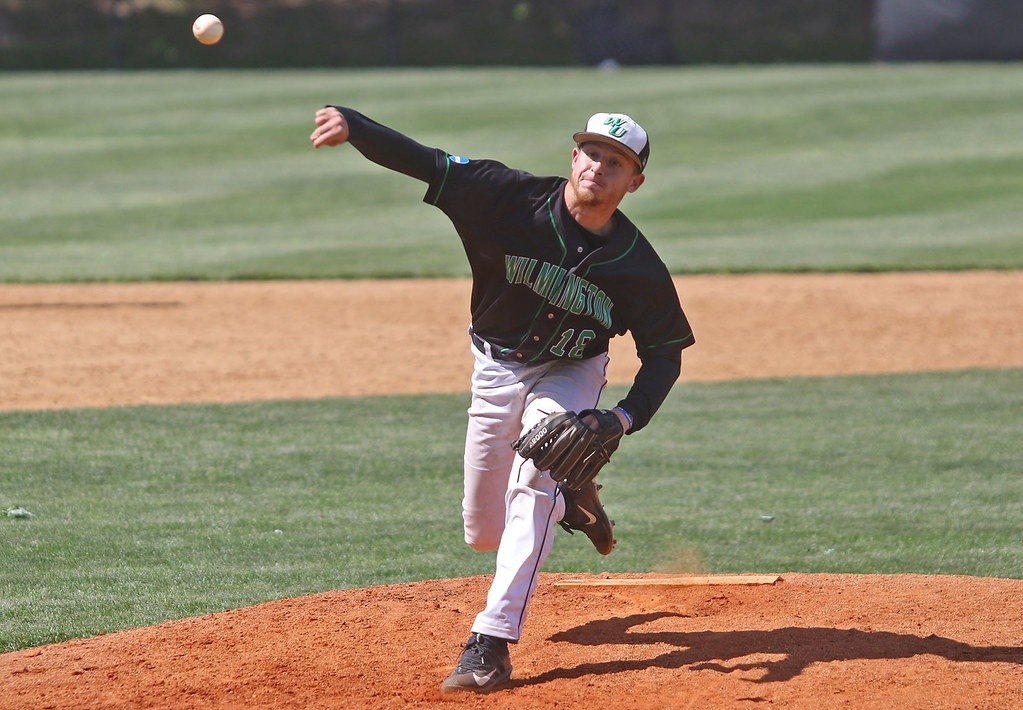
[468,327,515,361]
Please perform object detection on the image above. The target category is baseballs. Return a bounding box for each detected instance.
[192,13,224,45]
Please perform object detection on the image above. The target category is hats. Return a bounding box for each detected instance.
[573,113,650,173]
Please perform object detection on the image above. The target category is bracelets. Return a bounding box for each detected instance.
[613,406,633,429]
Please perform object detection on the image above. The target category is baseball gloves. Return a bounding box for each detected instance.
[508,407,626,502]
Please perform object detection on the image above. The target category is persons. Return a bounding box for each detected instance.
[310,105,696,693]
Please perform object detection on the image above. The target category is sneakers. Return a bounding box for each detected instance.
[442,634,512,693]
[556,480,617,555]
